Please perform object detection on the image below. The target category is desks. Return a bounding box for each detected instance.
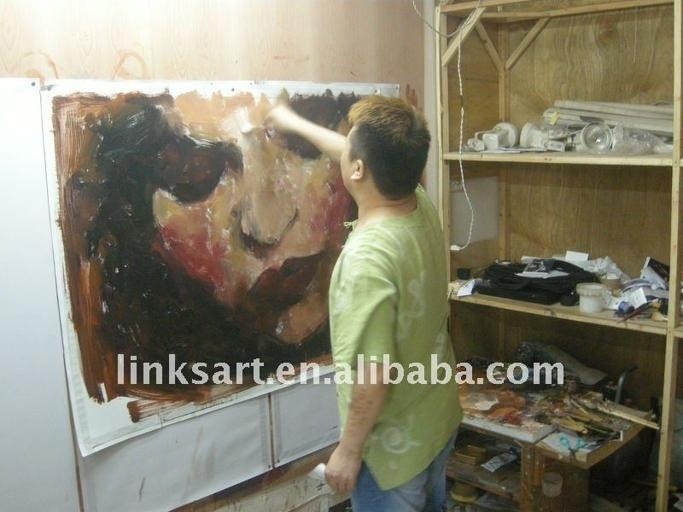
[445,366,651,512]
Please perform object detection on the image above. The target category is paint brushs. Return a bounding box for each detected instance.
[241,121,272,134]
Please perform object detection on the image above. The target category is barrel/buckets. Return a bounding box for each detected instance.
[649,397,683,486]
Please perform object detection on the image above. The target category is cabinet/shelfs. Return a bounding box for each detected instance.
[437,0,683,511]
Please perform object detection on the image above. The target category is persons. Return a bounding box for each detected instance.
[262,95,463,512]
